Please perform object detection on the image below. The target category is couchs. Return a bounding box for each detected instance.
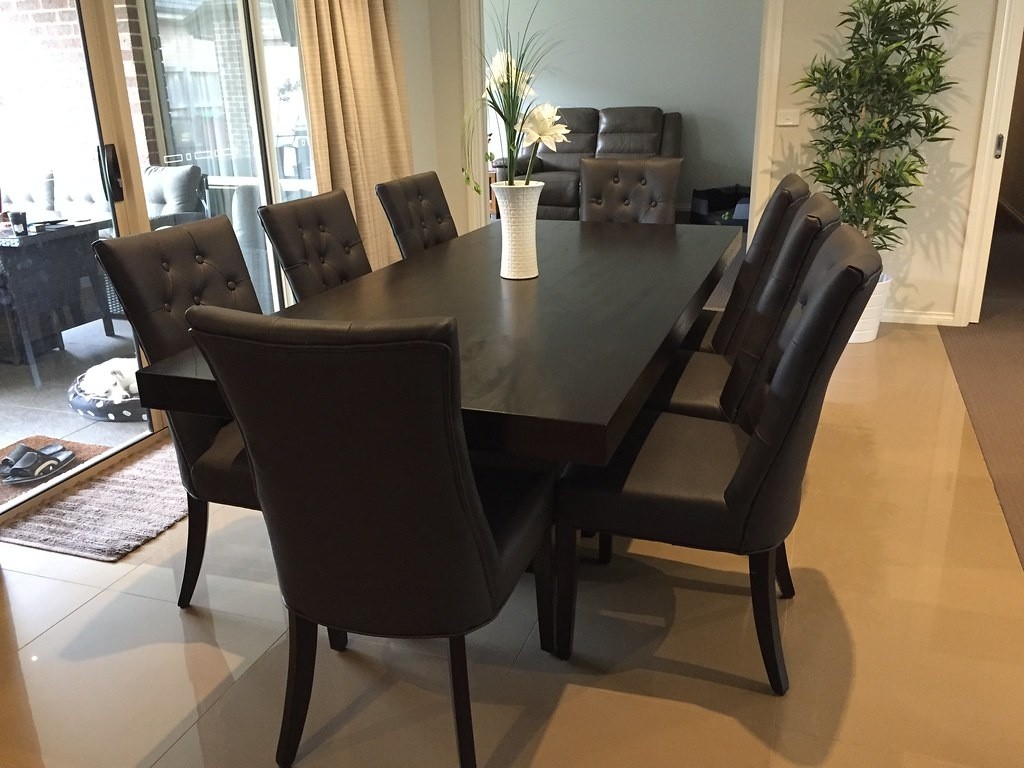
[497,107,682,221]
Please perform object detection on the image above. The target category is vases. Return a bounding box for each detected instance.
[490,180,546,280]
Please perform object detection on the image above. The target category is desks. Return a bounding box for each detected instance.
[135,219,743,659]
[0,217,116,389]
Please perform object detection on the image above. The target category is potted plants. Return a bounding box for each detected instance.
[790,0,959,344]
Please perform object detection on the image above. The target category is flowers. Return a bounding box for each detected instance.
[461,0,573,195]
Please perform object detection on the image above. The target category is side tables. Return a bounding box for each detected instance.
[488,171,500,219]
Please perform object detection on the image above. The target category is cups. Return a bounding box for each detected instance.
[7,211,28,237]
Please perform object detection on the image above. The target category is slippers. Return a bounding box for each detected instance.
[3,450,75,485]
[0,444,65,477]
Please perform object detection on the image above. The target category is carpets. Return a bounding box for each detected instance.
[0,435,112,505]
[0,434,187,563]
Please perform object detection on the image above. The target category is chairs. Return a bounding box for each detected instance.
[142,163,208,231]
[578,158,685,223]
[554,173,884,697]
[258,187,370,297]
[231,184,274,317]
[184,304,564,768]
[91,214,261,611]
[375,170,459,260]
